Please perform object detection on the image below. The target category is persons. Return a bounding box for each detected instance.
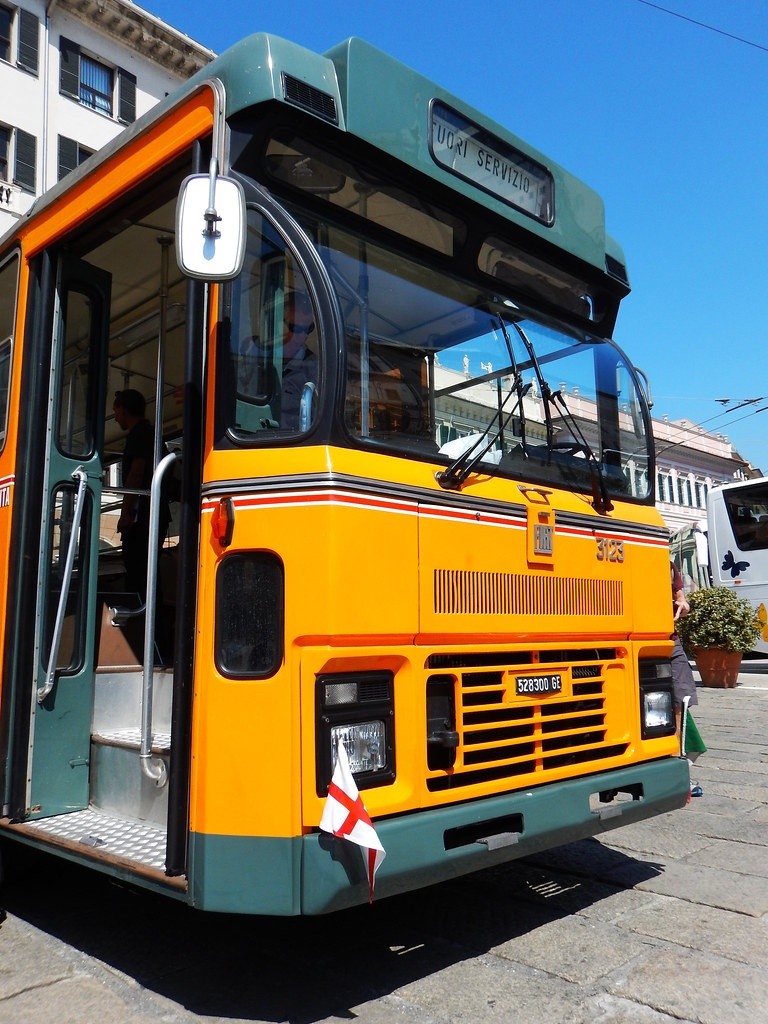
[110,387,172,622]
[241,290,321,428]
[670,560,703,796]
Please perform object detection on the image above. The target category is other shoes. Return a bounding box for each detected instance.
[690,786,703,797]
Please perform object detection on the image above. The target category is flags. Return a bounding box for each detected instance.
[681,695,707,767]
[318,737,385,903]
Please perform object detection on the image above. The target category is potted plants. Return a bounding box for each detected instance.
[674,587,767,688]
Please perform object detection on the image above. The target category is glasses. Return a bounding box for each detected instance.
[283,319,315,333]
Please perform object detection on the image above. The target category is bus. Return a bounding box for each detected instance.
[0,31,704,924]
[672,521,713,592]
[704,477,768,667]
